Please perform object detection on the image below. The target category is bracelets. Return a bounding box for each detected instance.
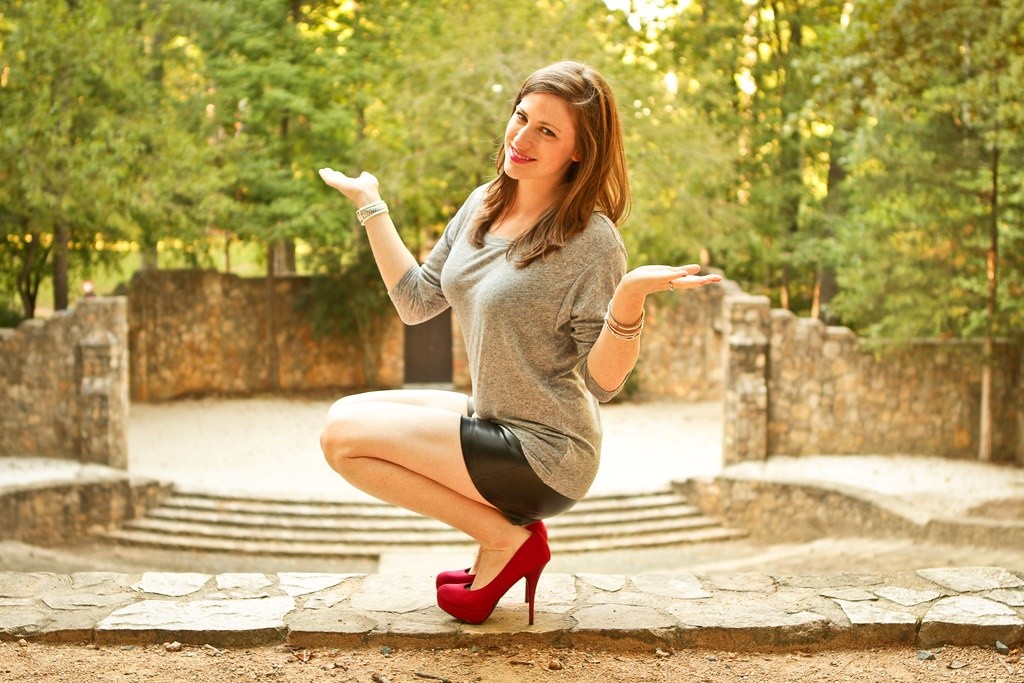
[356,200,388,225]
[604,299,645,340]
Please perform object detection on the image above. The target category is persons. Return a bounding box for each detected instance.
[320,62,721,624]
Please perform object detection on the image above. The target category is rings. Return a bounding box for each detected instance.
[669,281,674,291]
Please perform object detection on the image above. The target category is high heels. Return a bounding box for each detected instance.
[436,521,548,602]
[437,528,551,625]
